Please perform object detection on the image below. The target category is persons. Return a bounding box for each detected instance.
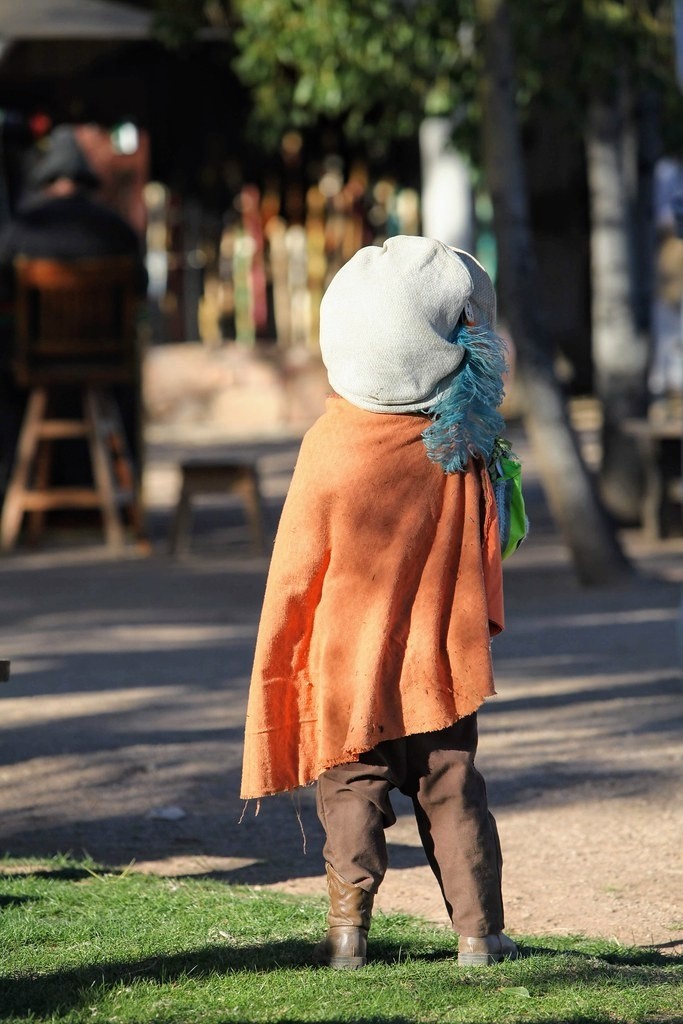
[240,233,519,972]
[4,131,152,496]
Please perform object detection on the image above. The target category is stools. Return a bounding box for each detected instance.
[0,258,144,551]
[621,418,683,539]
[165,458,267,553]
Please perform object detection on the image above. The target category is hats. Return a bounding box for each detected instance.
[26,125,98,188]
[318,234,511,476]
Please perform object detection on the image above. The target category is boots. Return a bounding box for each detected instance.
[457,933,518,966]
[312,861,375,971]
